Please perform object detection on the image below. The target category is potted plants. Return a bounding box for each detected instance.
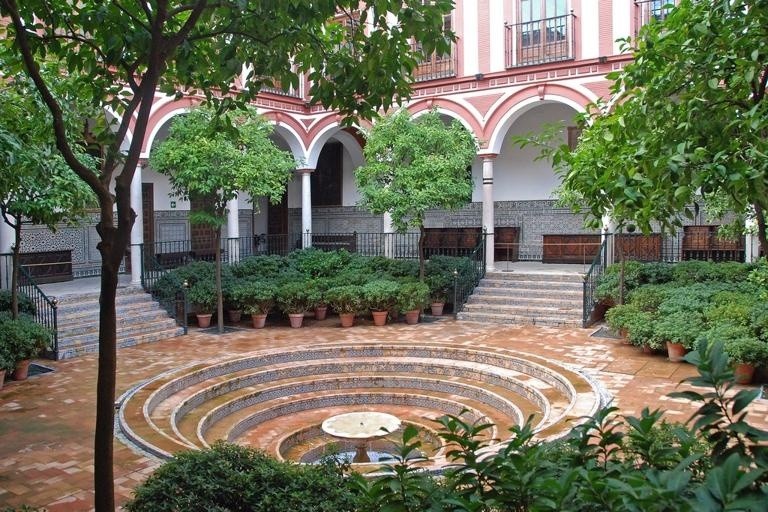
[596,256,767,385]
[157,248,480,329]
[0,319,57,389]
